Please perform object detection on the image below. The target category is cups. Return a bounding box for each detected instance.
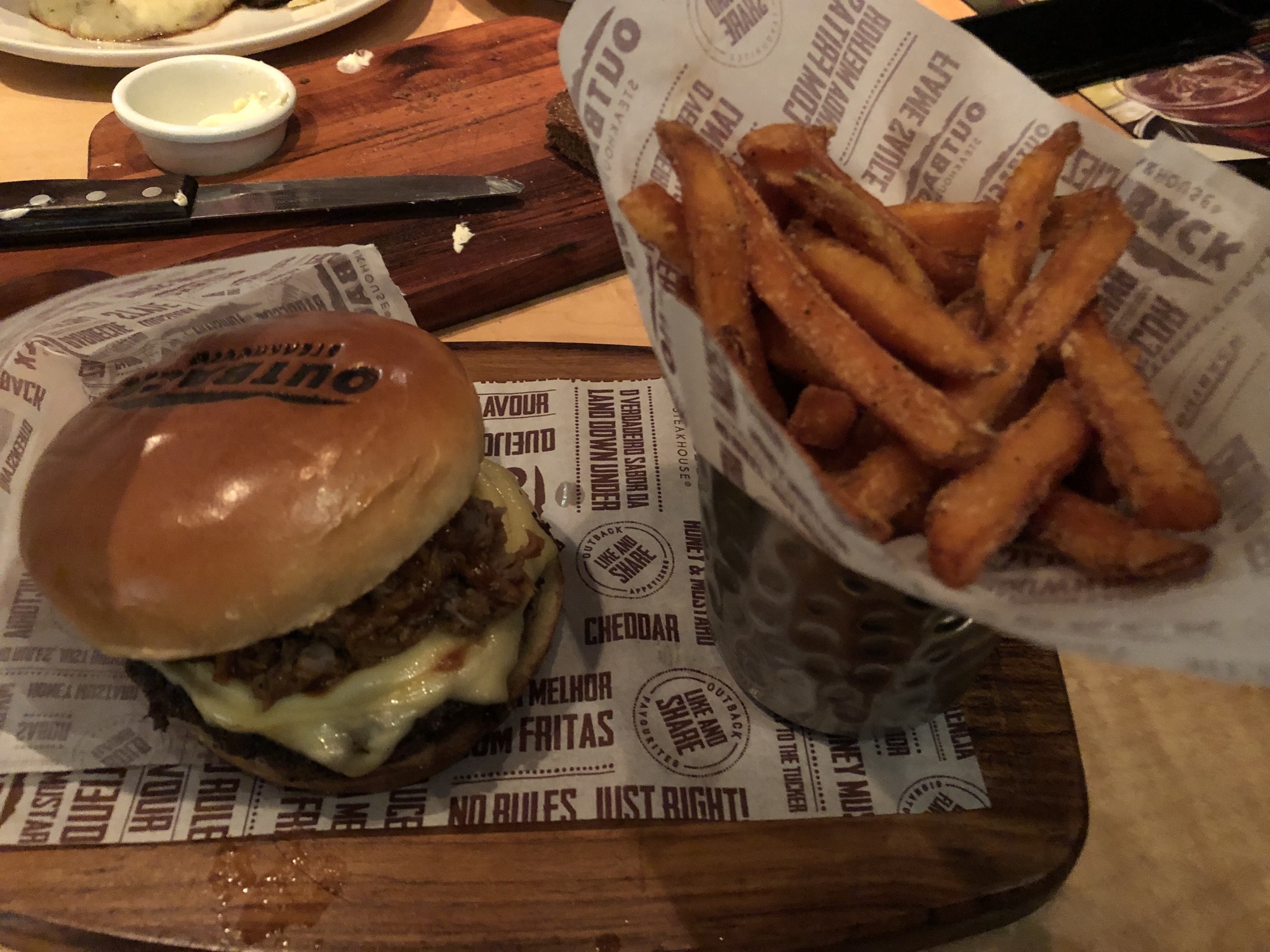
[692,441,1066,744]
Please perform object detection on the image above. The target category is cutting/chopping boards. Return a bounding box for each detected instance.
[0,11,632,383]
[2,302,1091,948]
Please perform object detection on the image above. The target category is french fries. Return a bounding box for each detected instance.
[617,118,1225,586]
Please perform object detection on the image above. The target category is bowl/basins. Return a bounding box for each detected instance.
[110,53,298,172]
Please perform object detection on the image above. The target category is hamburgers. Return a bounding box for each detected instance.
[21,309,568,794]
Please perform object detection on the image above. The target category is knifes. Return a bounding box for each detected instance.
[1,170,528,229]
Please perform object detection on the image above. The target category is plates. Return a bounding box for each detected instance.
[2,0,390,70]
